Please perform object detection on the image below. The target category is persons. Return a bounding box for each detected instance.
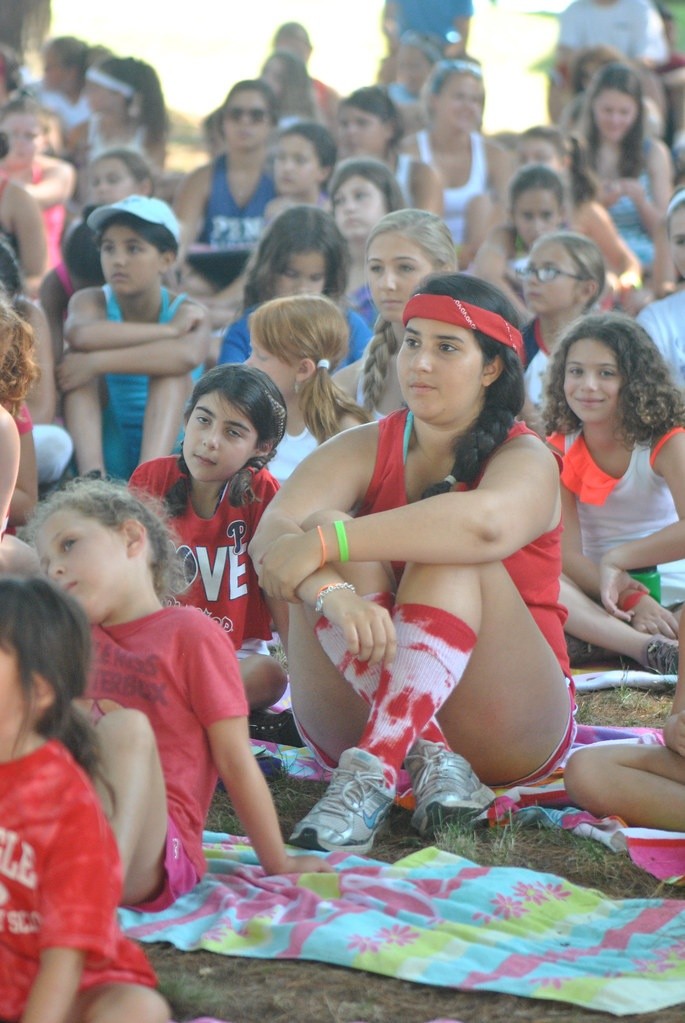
[218,206,374,373]
[564,600,685,833]
[129,364,308,749]
[39,205,109,415]
[332,209,457,421]
[243,294,371,482]
[0,578,174,1023]
[540,314,685,675]
[521,233,605,436]
[635,188,685,400]
[248,273,577,853]
[0,0,685,295]
[0,474,335,913]
[0,237,74,580]
[54,195,212,481]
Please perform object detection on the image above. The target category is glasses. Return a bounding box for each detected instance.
[223,107,266,122]
[516,266,586,284]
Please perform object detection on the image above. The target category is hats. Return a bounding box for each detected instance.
[87,195,182,247]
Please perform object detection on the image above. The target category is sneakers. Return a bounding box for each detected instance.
[642,633,680,676]
[403,737,498,842]
[287,746,398,856]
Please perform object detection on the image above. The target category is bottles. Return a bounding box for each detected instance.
[626,565,661,606]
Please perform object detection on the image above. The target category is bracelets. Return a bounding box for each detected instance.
[315,582,355,613]
[334,521,348,562]
[622,592,647,612]
[317,526,326,568]
